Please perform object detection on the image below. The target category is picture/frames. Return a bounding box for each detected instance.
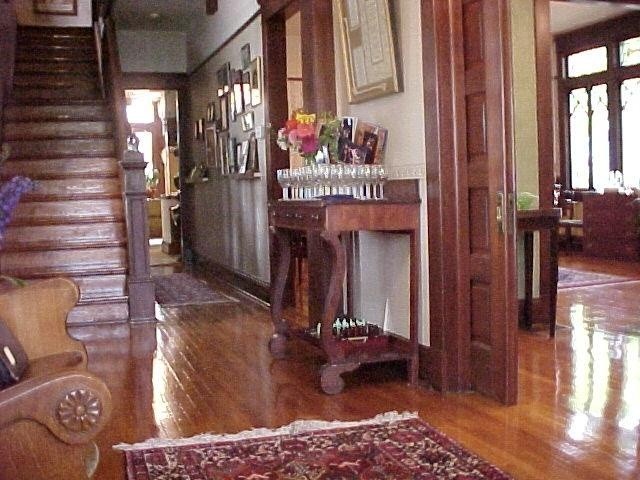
[187,43,262,173]
[336,0,404,104]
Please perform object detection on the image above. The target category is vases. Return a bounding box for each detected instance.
[304,157,315,166]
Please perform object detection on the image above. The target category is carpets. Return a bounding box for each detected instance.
[558,265,640,290]
[113,411,514,480]
[151,272,241,307]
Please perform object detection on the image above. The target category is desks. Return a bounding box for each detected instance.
[517,207,564,338]
[267,179,423,392]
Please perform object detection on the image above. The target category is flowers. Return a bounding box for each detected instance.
[267,105,342,162]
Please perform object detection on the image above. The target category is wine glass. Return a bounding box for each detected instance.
[277,164,387,200]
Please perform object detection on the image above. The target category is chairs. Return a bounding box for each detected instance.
[0,276,114,480]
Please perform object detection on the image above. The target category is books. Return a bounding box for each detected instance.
[323,115,392,167]
